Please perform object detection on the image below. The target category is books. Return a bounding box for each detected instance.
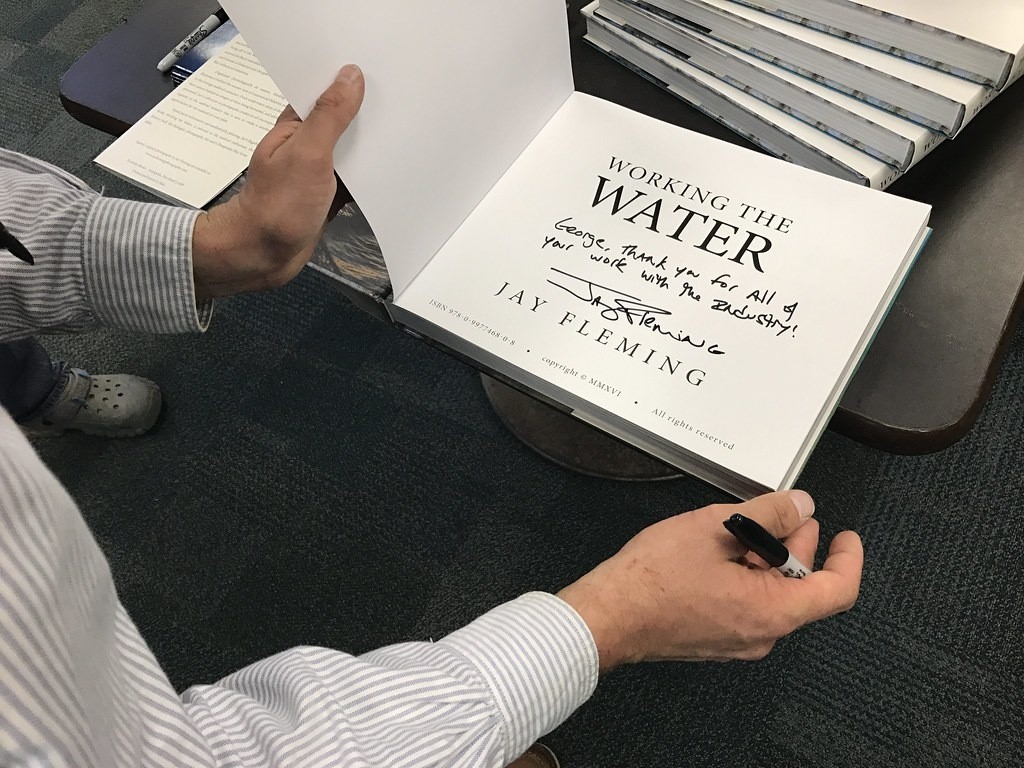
[91,0,930,504]
[579,0,1023,191]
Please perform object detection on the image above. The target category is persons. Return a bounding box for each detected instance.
[0,338,163,440]
[0,63,864,767]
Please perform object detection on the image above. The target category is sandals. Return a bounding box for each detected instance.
[20,367,162,435]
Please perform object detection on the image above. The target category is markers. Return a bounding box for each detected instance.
[722,513,810,578]
[157,6,228,72]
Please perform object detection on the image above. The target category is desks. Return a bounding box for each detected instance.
[57,1,1024,485]
